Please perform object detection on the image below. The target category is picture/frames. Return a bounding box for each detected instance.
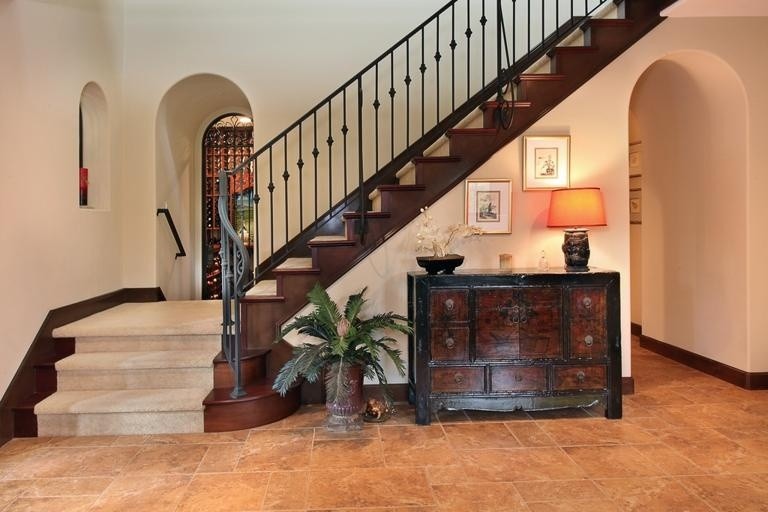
[462,177,513,236]
[629,140,642,179]
[629,188,642,225]
[230,184,253,252]
[520,132,572,193]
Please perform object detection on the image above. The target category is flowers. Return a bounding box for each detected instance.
[413,205,487,258]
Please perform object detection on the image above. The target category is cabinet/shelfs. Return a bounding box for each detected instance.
[405,266,630,425]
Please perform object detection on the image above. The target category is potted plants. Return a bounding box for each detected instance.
[272,279,415,429]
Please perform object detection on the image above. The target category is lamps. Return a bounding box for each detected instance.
[547,186,609,271]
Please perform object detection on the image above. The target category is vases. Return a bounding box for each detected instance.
[416,253,465,275]
